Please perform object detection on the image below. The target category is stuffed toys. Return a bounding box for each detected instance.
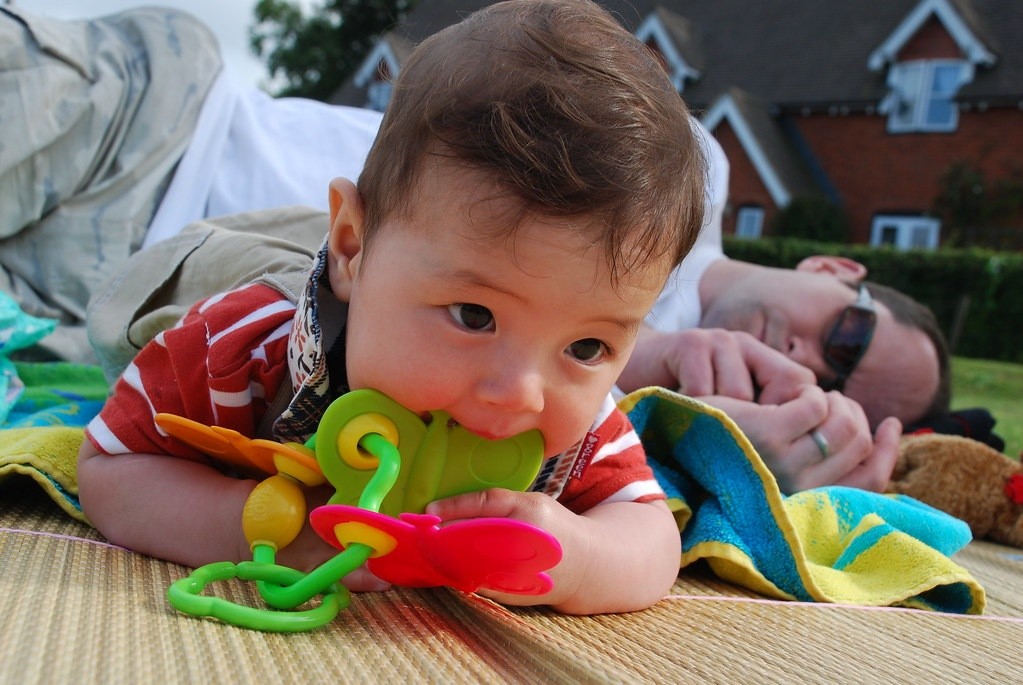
[889,434,1023,548]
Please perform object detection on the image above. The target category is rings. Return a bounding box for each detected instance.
[811,428,832,459]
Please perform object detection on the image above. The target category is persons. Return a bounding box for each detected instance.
[78,0,707,614]
[0,0,952,495]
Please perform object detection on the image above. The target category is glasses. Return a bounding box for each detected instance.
[810,283,877,393]
